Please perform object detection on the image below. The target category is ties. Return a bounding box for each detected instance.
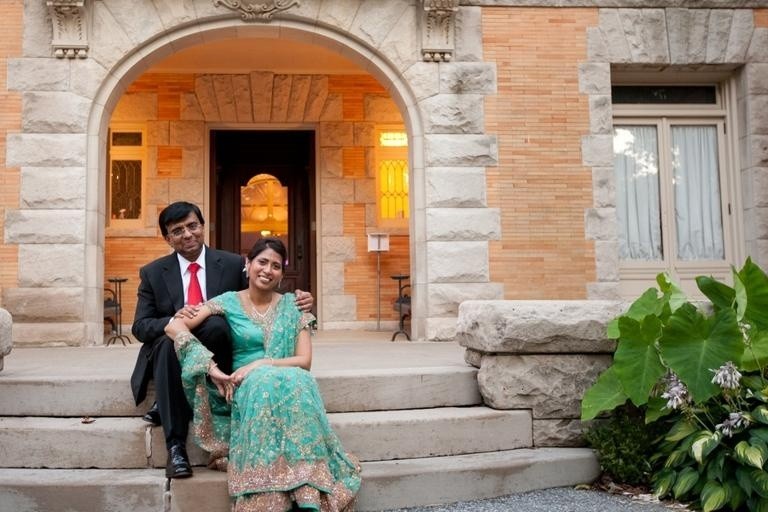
[188,263,203,305]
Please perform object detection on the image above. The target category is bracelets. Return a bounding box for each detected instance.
[206,363,220,377]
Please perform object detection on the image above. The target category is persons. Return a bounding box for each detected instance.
[130,201,312,478]
[164,237,361,512]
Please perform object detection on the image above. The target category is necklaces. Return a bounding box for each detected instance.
[244,287,273,324]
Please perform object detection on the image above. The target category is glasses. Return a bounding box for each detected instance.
[166,224,201,237]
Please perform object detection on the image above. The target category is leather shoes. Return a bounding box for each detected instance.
[143,401,160,426]
[166,445,193,478]
